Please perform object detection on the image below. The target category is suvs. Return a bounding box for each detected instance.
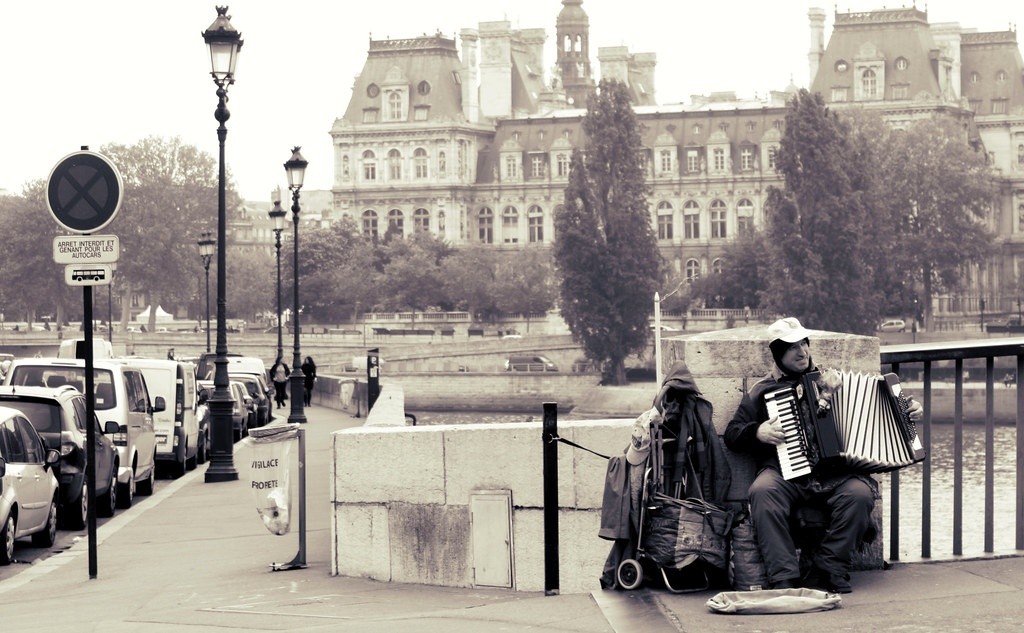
[0,383,122,532]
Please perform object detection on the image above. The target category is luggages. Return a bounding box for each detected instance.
[617,422,737,593]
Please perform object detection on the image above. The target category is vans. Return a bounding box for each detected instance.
[505,354,559,372]
[3,356,167,509]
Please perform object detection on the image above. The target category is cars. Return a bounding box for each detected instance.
[875,318,905,333]
[0,405,62,565]
[0,331,276,565]
[595,367,656,385]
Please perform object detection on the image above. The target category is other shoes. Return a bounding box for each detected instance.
[773,575,802,590]
[281,400,285,407]
[277,407,280,409]
[813,568,852,594]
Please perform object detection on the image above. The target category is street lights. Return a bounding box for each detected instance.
[200,5,246,484]
[269,200,286,364]
[283,144,307,423]
[197,231,216,353]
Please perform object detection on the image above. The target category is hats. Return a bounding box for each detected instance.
[625,407,661,466]
[767,316,810,345]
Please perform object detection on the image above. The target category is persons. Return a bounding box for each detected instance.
[269,356,290,409]
[301,356,317,407]
[723,317,924,594]
